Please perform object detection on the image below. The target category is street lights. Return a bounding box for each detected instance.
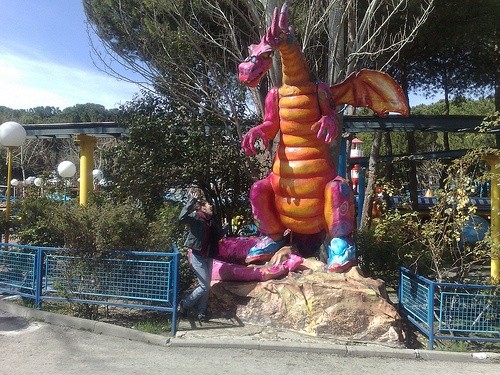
[0,121,26,252]
[25,176,42,196]
[58,161,77,204]
[10,178,19,199]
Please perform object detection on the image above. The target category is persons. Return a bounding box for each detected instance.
[179,192,231,322]
[425,187,434,197]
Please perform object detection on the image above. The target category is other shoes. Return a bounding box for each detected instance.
[197,313,206,321]
[177,302,186,319]
